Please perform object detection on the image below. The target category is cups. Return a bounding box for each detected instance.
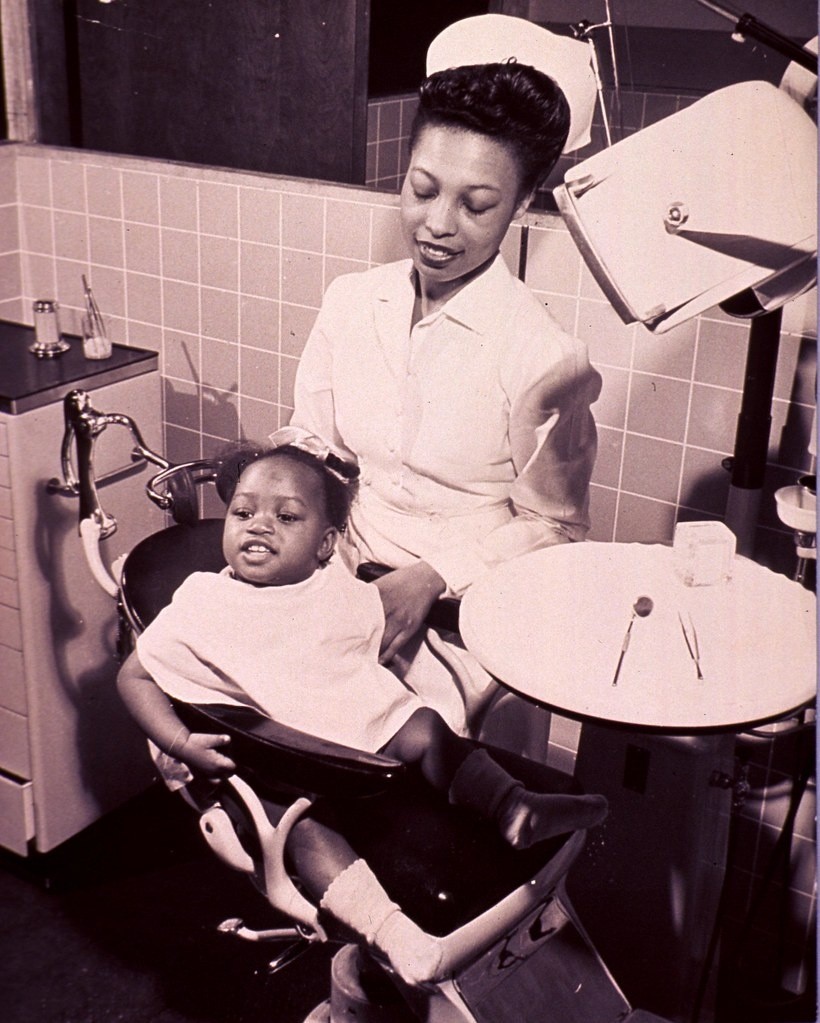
[672,520,737,586]
[81,314,113,360]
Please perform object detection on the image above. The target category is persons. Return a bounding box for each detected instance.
[268,62,603,737]
[116,446,609,985]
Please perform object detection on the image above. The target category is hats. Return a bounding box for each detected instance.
[425,12,599,155]
